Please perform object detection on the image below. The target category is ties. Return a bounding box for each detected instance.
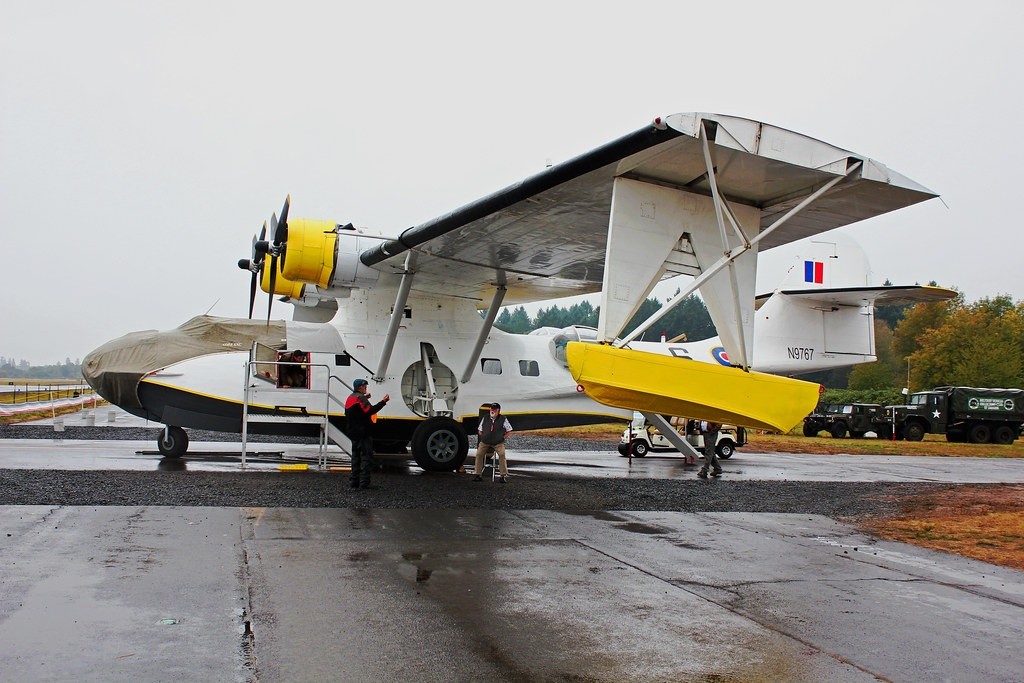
[491,419,494,432]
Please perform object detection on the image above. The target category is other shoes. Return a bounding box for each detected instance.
[359,483,374,489]
[710,468,723,477]
[350,483,360,489]
[471,475,483,481]
[697,470,708,479]
[499,477,507,483]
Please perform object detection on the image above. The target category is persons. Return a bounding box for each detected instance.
[472,403,513,482]
[345,379,389,489]
[279,350,305,389]
[697,420,723,478]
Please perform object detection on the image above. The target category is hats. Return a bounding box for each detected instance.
[353,379,368,387]
[489,403,500,408]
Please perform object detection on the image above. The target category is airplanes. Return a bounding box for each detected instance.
[80,112,962,478]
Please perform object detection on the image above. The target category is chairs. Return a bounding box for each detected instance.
[654,416,685,436]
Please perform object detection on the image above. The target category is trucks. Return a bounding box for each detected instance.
[883,385,1024,445]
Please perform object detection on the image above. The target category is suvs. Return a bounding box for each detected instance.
[802,401,889,439]
